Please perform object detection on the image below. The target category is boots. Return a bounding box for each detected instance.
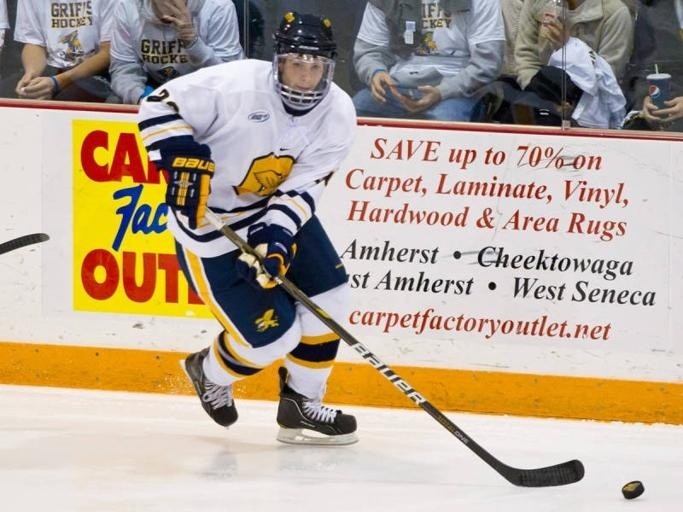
[278,367,356,435]
[185,349,237,426]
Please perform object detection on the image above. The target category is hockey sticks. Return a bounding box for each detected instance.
[204,205,583,486]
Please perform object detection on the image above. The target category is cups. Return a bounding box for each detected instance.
[538,1,569,40]
[647,74,673,118]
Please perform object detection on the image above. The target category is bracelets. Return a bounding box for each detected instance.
[182,37,198,49]
[50,75,58,97]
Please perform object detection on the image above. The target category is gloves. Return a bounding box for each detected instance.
[166,144,215,229]
[235,222,297,289]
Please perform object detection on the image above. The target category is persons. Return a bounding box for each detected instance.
[350,1,508,123]
[0,0,9,52]
[14,1,113,103]
[135,12,365,437]
[104,0,248,106]
[470,0,635,129]
[620,1,682,132]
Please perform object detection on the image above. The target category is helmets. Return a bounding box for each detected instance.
[272,12,337,111]
